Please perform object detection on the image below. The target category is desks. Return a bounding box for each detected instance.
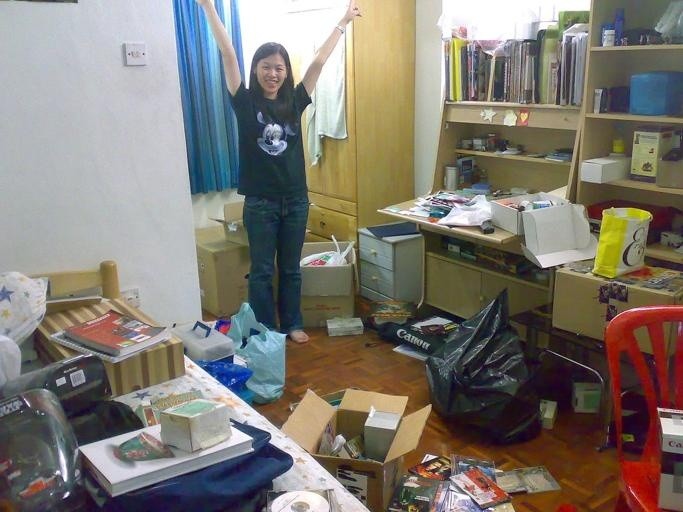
[373,102,579,247]
[502,299,678,452]
[105,355,371,512]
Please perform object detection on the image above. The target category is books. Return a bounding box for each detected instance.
[388,451,562,512]
[49,332,172,363]
[446,10,590,106]
[62,309,169,358]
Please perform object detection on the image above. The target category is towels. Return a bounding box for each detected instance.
[288,9,348,170]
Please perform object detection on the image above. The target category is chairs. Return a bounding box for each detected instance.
[603,306,682,510]
[26,260,123,303]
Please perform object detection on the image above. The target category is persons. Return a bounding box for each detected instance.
[197,1,362,342]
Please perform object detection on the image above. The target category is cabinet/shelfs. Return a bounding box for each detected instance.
[549,1,683,264]
[424,254,554,348]
[284,2,416,245]
[351,218,424,305]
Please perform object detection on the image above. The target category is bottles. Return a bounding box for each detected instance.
[613,8,625,46]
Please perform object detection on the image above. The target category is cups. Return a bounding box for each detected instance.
[612,140,627,154]
[443,166,460,193]
[601,25,614,48]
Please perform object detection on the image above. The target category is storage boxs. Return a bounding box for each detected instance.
[273,382,432,510]
[275,237,357,327]
[626,68,682,116]
[170,322,237,365]
[579,125,683,188]
[549,260,683,358]
[209,200,254,249]
[487,190,597,270]
[33,296,186,395]
[650,406,680,510]
[191,219,312,319]
[288,384,366,416]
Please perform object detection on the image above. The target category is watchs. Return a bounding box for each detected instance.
[335,25,346,34]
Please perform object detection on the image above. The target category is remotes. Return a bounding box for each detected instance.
[480,222,494,233]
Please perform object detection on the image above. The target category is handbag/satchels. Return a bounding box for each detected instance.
[593,207,653,279]
